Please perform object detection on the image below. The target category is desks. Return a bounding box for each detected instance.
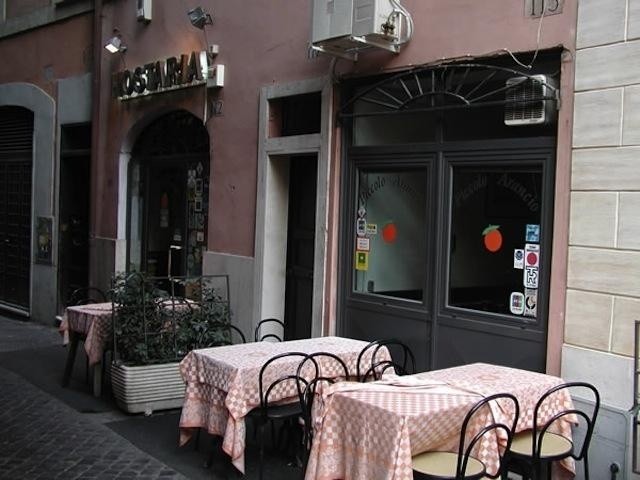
[305,358,581,480]
[174,334,397,479]
[60,297,200,400]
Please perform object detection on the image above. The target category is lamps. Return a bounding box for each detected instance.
[187,6,216,31]
[104,27,129,57]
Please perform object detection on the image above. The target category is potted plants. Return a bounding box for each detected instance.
[110,266,240,418]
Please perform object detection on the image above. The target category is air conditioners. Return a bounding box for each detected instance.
[304,0,415,65]
[504,73,557,126]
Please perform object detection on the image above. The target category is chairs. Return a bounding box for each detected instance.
[508,378,604,479]
[251,350,322,479]
[65,286,108,383]
[410,388,521,480]
[193,323,246,470]
[352,338,420,384]
[252,318,292,345]
[292,351,350,479]
